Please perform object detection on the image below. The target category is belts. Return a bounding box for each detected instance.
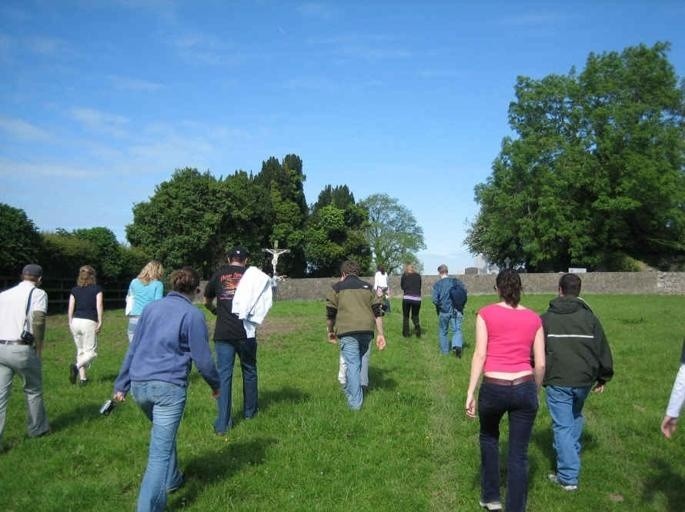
[482,374,534,388]
[1,339,28,346]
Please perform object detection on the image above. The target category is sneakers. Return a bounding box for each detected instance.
[78,379,89,387]
[453,347,463,359]
[68,364,78,383]
[479,498,501,510]
[548,474,578,490]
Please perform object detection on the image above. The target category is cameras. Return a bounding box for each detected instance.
[21,331,37,347]
[100,399,116,416]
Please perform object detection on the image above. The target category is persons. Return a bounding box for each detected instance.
[465,270,546,511]
[370,264,390,315]
[0,264,52,453]
[659,339,685,440]
[324,260,386,410]
[205,244,276,433]
[537,274,615,490]
[433,264,469,359]
[113,268,221,512]
[125,260,165,346]
[400,264,424,340]
[334,272,370,393]
[66,264,103,385]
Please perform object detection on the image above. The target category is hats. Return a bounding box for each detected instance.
[22,264,44,277]
[230,248,247,258]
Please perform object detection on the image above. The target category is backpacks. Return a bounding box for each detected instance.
[448,279,466,316]
